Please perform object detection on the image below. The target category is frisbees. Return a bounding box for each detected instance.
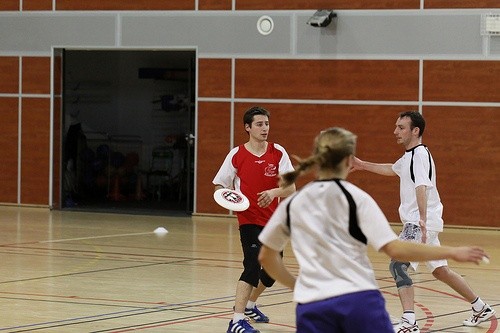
[212,187,251,213]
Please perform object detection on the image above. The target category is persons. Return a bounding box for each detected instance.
[350,110,496,333]
[62,124,181,202]
[258,125,490,333]
[211,107,296,333]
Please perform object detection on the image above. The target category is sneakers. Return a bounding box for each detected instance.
[227,320,260,333]
[243,305,269,323]
[394,318,422,333]
[463,304,496,326]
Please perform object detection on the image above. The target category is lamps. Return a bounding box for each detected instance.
[306,9,337,27]
